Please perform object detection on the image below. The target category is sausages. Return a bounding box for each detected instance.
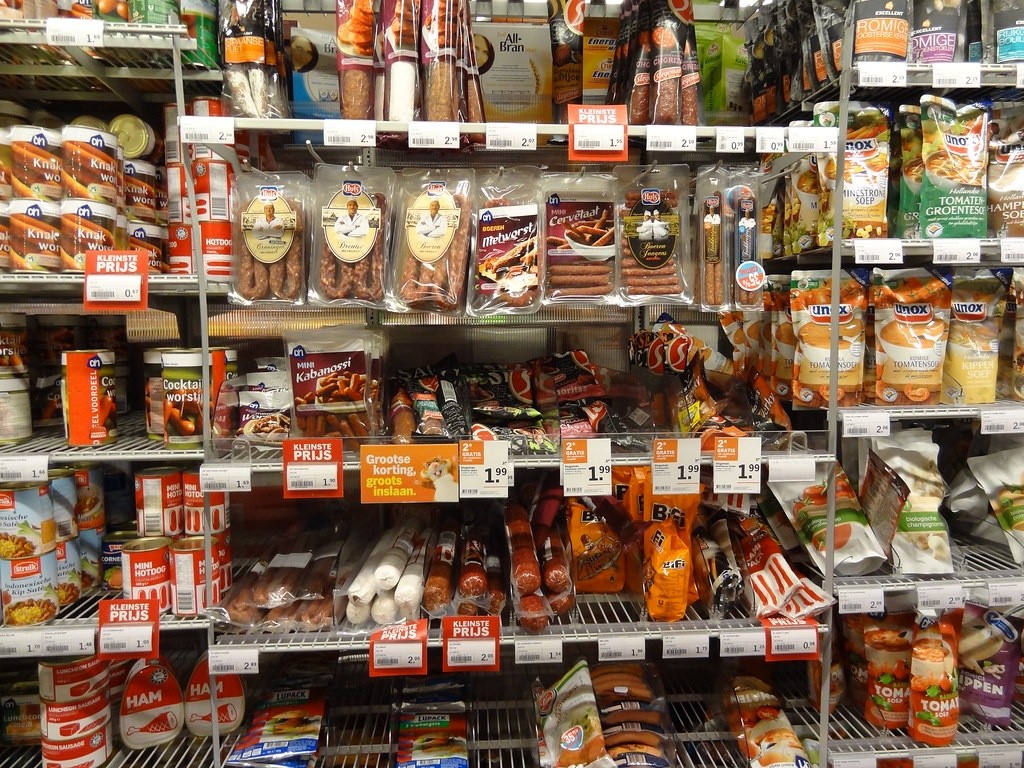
[209,0,794,768]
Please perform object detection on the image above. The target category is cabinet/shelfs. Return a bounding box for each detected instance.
[0,17,1024,768]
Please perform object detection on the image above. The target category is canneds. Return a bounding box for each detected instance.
[0,0,235,768]
